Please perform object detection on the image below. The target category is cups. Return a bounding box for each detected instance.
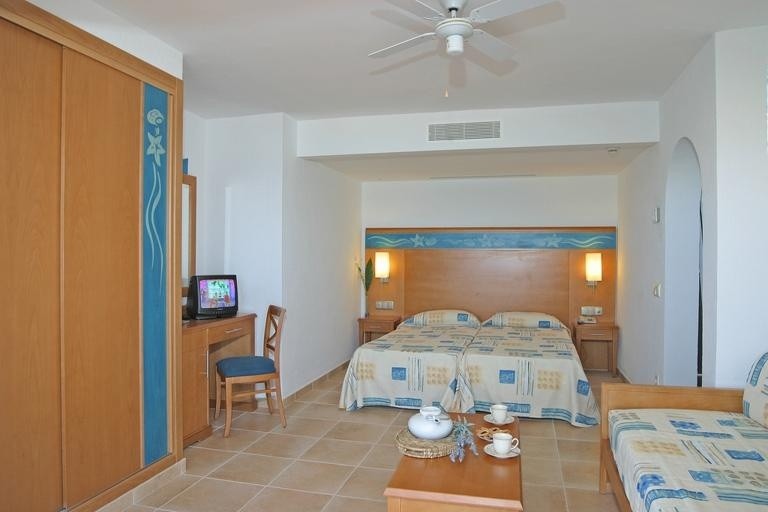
[493,433,519,454]
[490,405,508,422]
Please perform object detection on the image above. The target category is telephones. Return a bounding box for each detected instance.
[577,315,598,324]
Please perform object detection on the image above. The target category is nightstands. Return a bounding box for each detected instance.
[357,315,401,345]
[572,322,620,378]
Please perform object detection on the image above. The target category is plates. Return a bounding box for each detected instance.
[483,444,521,458]
[483,414,515,425]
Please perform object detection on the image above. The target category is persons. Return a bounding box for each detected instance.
[207,291,230,308]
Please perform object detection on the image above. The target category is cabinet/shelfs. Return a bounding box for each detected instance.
[181,313,258,449]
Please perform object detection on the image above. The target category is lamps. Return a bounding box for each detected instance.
[585,252,602,285]
[374,251,390,284]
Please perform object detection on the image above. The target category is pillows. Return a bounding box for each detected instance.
[400,310,481,328]
[481,311,570,329]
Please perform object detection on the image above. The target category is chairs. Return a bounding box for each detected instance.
[214,305,286,437]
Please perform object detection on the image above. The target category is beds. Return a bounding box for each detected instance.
[339,309,482,412]
[599,353,768,512]
[462,311,601,427]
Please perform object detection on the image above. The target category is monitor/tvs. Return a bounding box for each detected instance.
[185,273,240,319]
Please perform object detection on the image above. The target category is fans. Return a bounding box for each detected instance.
[369,0,557,63]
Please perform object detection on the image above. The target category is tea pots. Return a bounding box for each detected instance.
[407,406,453,439]
